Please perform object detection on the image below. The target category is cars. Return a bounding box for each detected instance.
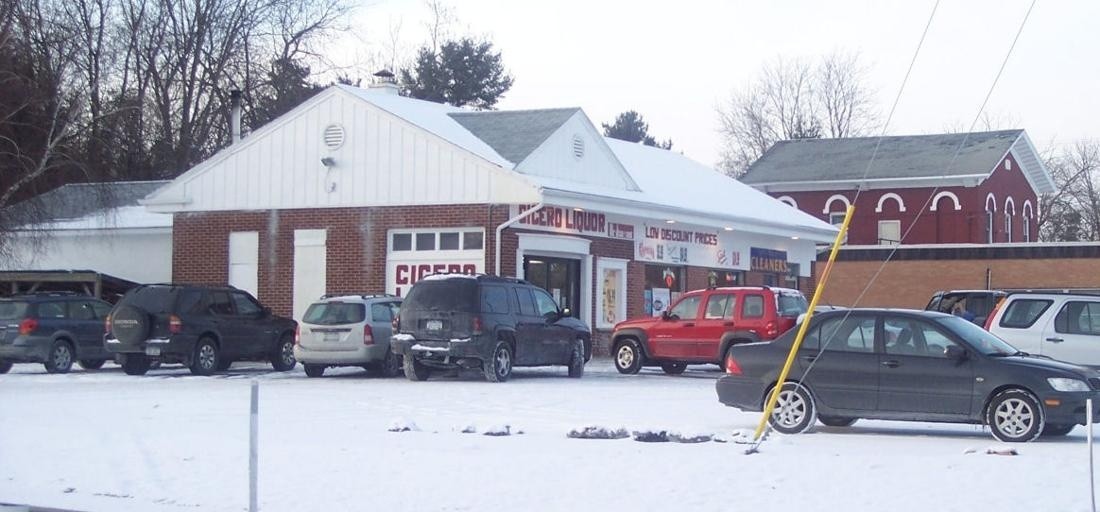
[292,272,591,383]
[0,291,114,374]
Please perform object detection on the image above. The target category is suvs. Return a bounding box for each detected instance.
[103,282,300,375]
